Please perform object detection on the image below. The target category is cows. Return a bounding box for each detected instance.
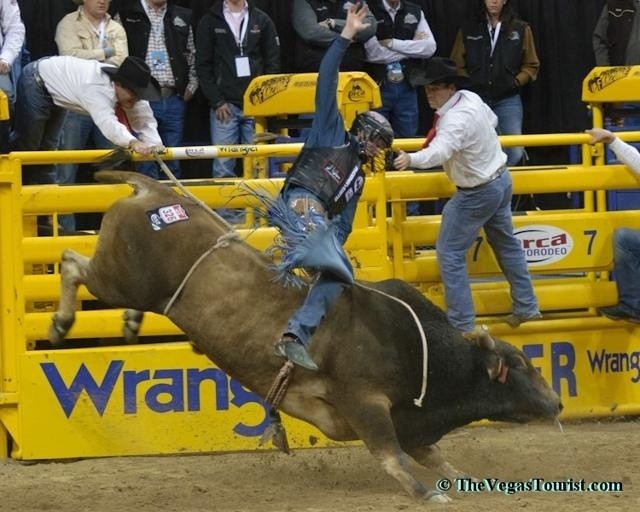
[48,146,564,503]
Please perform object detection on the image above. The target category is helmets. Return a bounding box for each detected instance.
[352,112,394,163]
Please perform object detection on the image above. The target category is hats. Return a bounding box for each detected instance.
[410,57,470,86]
[100,56,162,101]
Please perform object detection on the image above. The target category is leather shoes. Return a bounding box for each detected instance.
[273,340,319,371]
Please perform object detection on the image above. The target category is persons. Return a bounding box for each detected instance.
[583,126,640,326]
[394,55,543,336]
[0,0,640,231]
[273,3,397,372]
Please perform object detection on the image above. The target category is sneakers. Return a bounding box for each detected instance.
[598,306,640,323]
[504,314,544,327]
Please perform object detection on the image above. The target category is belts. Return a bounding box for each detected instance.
[31,53,48,93]
[491,164,506,181]
